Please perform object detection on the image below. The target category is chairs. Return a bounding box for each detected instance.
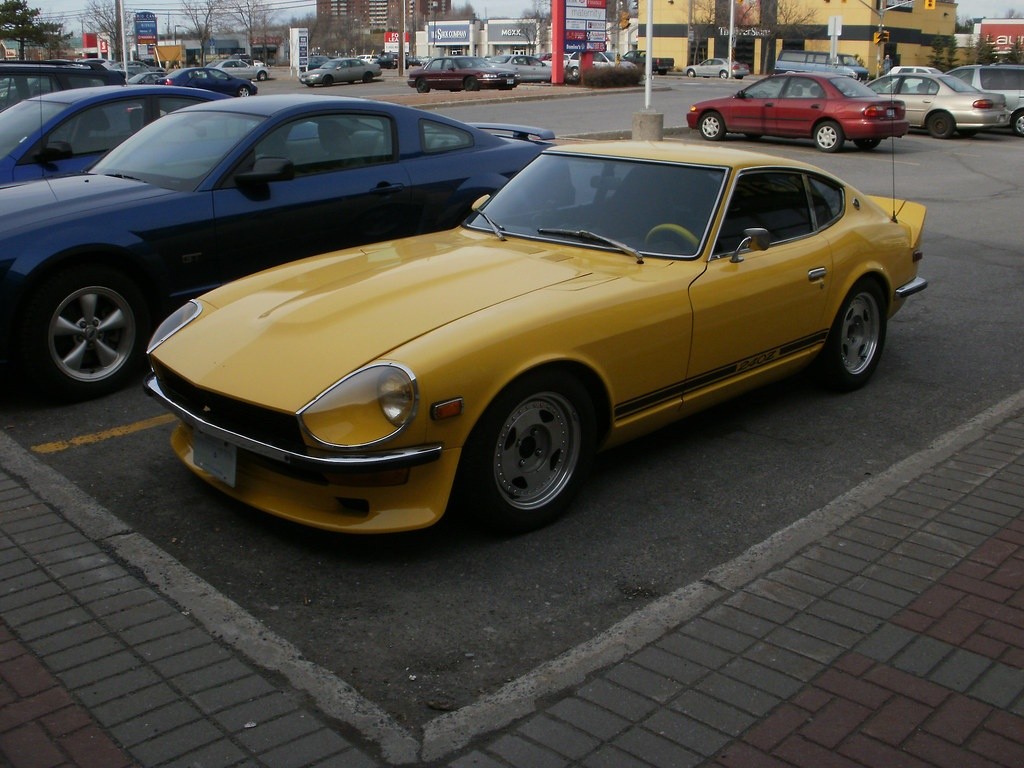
[315,119,366,169]
[130,108,146,133]
[792,85,821,97]
[83,108,112,149]
[899,84,928,93]
[261,131,293,165]
[579,165,810,249]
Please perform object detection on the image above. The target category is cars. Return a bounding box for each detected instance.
[524,50,638,81]
[0,84,319,188]
[81,57,170,85]
[885,64,943,88]
[303,50,431,71]
[685,70,910,153]
[299,57,384,87]
[407,56,523,94]
[206,53,271,83]
[0,94,574,404]
[851,73,1011,139]
[155,67,258,97]
[484,55,554,85]
[682,58,750,80]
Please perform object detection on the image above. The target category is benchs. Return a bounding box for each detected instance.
[353,128,459,152]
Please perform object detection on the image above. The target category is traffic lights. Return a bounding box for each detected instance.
[874,0,936,45]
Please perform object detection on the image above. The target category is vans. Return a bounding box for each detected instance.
[774,50,870,83]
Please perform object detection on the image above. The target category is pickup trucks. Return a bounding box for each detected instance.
[621,48,676,76]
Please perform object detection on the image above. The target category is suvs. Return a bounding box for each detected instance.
[0,56,156,162]
[912,62,1024,137]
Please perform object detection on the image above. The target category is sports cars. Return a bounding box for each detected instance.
[145,141,930,535]
